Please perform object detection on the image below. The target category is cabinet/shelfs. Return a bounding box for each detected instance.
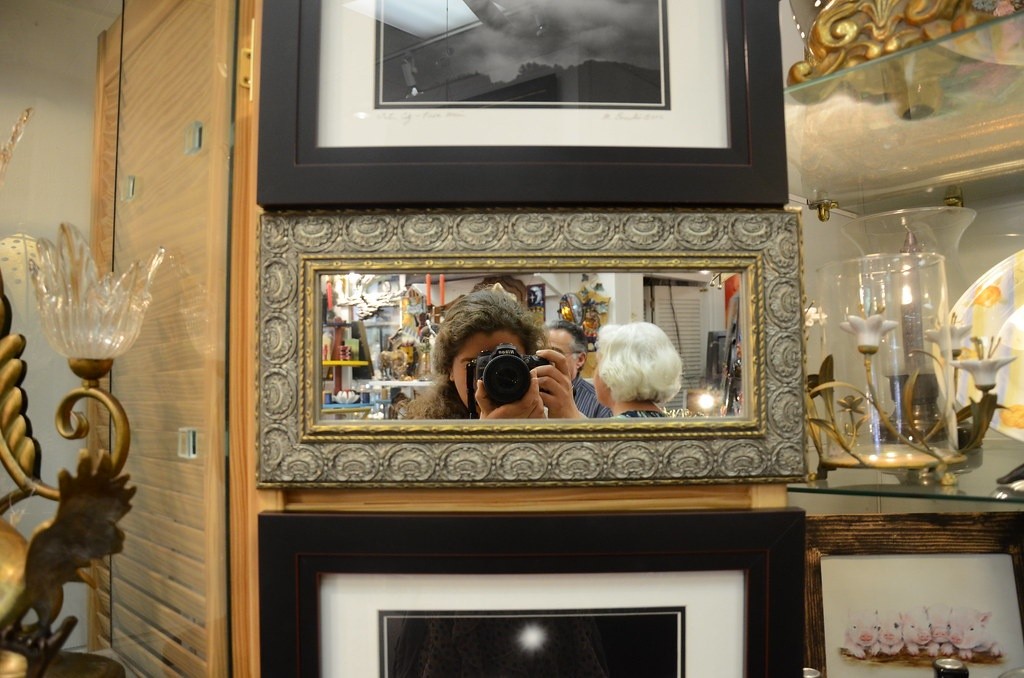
[784,11,1024,504]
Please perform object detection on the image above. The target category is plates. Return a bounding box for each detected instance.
[931,248,1024,445]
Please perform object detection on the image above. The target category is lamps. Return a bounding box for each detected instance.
[787,201,1020,490]
[0,107,166,678]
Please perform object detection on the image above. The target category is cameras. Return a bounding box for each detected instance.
[476,342,552,408]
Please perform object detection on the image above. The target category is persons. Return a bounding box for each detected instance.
[592,322,684,417]
[397,288,589,419]
[542,318,614,418]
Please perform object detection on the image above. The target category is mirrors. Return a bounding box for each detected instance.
[252,208,814,489]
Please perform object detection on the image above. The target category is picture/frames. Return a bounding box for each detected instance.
[254,507,808,678]
[804,512,1024,678]
[257,0,790,212]
